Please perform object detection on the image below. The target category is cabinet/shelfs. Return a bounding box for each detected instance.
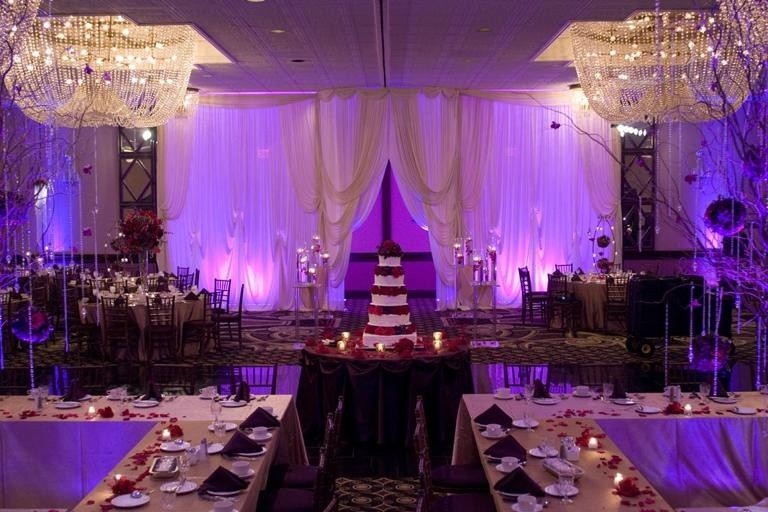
[294,257,330,343]
[456,260,502,344]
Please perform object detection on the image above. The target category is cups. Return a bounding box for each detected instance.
[207,386,218,395]
[118,386,129,396]
[27,389,40,398]
[198,387,210,396]
[518,495,536,512]
[495,387,591,394]
[106,388,117,397]
[262,406,274,415]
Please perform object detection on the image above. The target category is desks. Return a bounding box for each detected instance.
[1,391,299,512]
[289,325,479,488]
[450,392,767,512]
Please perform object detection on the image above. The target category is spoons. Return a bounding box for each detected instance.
[541,497,551,509]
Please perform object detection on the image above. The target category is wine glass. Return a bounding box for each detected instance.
[602,383,615,403]
[559,469,577,507]
[699,383,712,406]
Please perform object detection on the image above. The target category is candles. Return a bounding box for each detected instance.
[334,330,445,353]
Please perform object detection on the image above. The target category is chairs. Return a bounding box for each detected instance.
[2,257,245,363]
[504,361,768,396]
[410,397,495,512]
[3,362,279,395]
[518,258,767,358]
[258,394,348,511]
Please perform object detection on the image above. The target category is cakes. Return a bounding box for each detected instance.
[362,237,418,347]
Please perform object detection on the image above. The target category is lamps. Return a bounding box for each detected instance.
[563,0,768,129]
[714,0,768,69]
[0,0,42,74]
[168,85,202,120]
[0,12,199,131]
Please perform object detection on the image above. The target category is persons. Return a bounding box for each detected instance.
[711,222,751,346]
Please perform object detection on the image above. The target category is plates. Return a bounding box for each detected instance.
[493,393,757,415]
[511,503,543,512]
[109,409,280,511]
[56,394,255,409]
[473,418,580,500]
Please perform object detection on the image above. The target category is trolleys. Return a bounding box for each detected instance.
[625,275,735,356]
